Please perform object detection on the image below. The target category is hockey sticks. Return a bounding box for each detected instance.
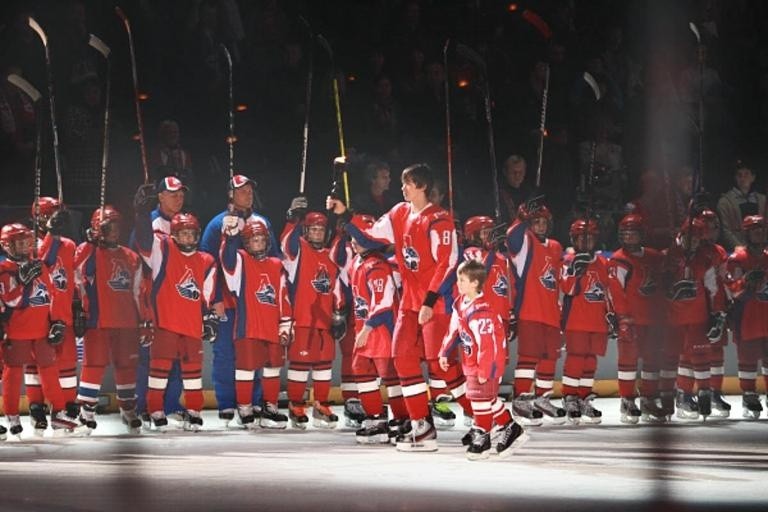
[28,17,63,213]
[583,70,601,251]
[298,24,317,195]
[218,40,235,214]
[116,7,150,186]
[687,20,703,204]
[318,34,351,212]
[444,28,454,220]
[536,60,549,196]
[87,33,116,225]
[9,75,43,264]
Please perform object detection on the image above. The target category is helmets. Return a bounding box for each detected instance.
[517,201,648,247]
[0,196,201,261]
[241,212,375,259]
[675,208,768,253]
[462,215,496,250]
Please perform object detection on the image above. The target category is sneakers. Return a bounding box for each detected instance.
[219,393,456,443]
[461,390,602,453]
[0,402,203,434]
[620,388,768,416]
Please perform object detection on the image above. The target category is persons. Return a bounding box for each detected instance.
[0,1,768,453]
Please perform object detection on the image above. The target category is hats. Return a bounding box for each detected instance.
[226,175,257,192]
[156,176,190,194]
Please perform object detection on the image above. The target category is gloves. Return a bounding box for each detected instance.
[705,311,727,344]
[136,319,156,348]
[48,300,87,344]
[333,309,348,339]
[18,262,42,286]
[278,317,296,345]
[203,308,218,342]
